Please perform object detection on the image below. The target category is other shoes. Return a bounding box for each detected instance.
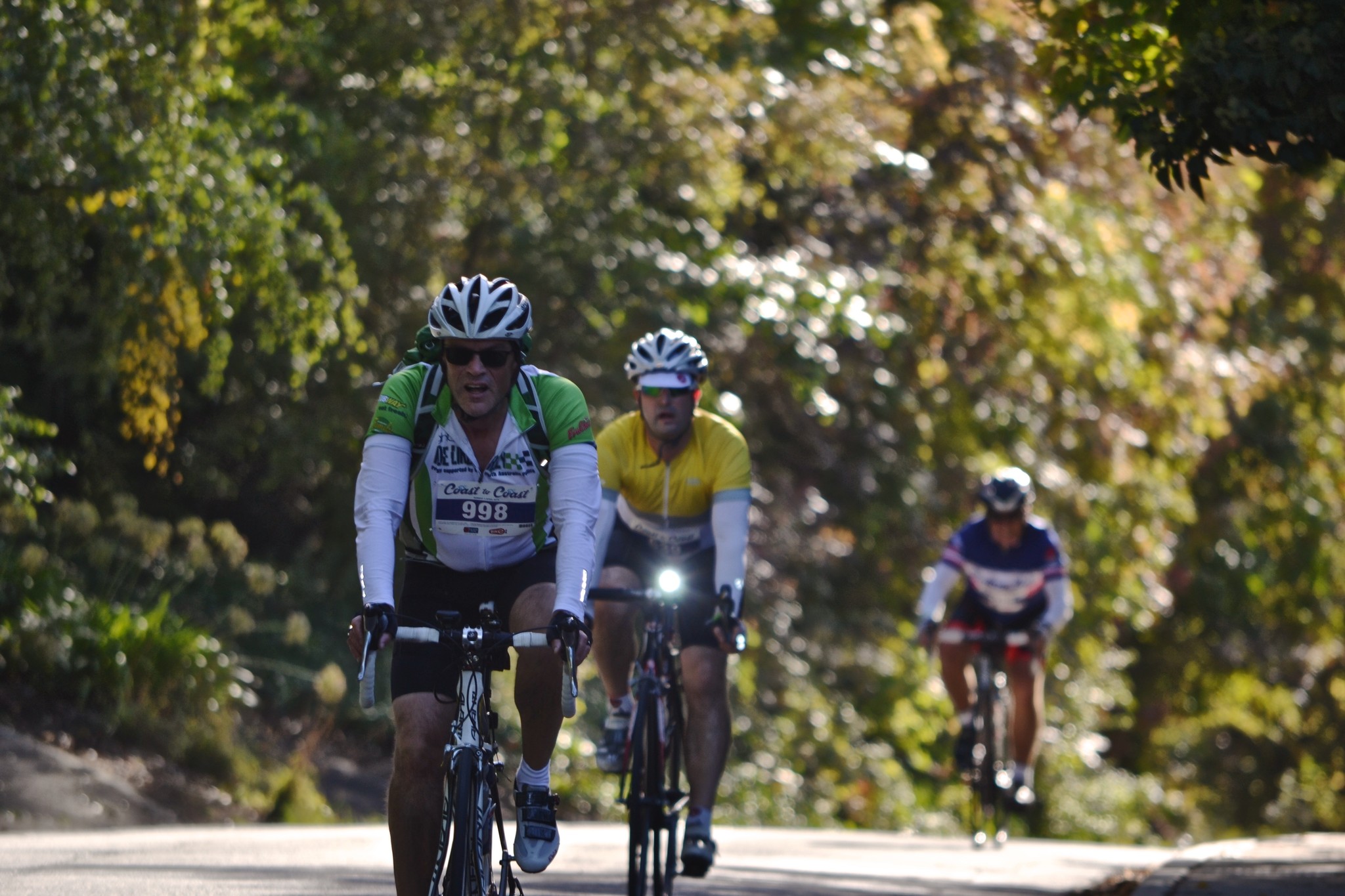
[956,720,982,771]
[1012,768,1035,804]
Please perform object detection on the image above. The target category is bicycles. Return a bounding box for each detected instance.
[911,627,1026,847]
[352,599,577,896]
[584,571,739,896]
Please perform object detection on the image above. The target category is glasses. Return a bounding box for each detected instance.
[642,385,686,398]
[441,344,519,370]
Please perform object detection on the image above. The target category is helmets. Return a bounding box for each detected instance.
[627,328,709,381]
[428,272,534,340]
[983,468,1036,520]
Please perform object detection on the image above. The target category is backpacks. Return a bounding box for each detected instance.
[391,325,551,479]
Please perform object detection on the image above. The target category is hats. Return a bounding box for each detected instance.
[636,374,695,389]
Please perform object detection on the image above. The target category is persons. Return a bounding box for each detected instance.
[348,275,600,896]
[596,327,751,877]
[916,471,1072,809]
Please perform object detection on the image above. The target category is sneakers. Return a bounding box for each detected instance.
[595,701,635,771]
[512,777,559,875]
[680,833,713,877]
